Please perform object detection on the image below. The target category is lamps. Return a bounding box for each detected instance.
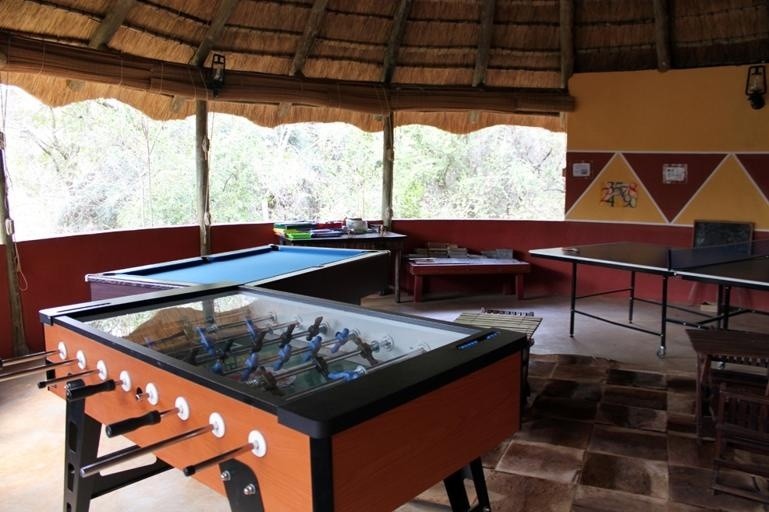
[745,65,767,109]
[206,53,225,98]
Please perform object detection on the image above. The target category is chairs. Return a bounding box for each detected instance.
[707,383,769,505]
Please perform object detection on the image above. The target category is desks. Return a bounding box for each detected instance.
[527,242,769,360]
[685,329,769,462]
[84,243,392,306]
[405,256,530,303]
[280,231,408,303]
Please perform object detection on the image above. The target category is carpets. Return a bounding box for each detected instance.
[394,352,769,512]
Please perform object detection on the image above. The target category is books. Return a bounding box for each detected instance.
[272,221,343,240]
[405,240,497,264]
